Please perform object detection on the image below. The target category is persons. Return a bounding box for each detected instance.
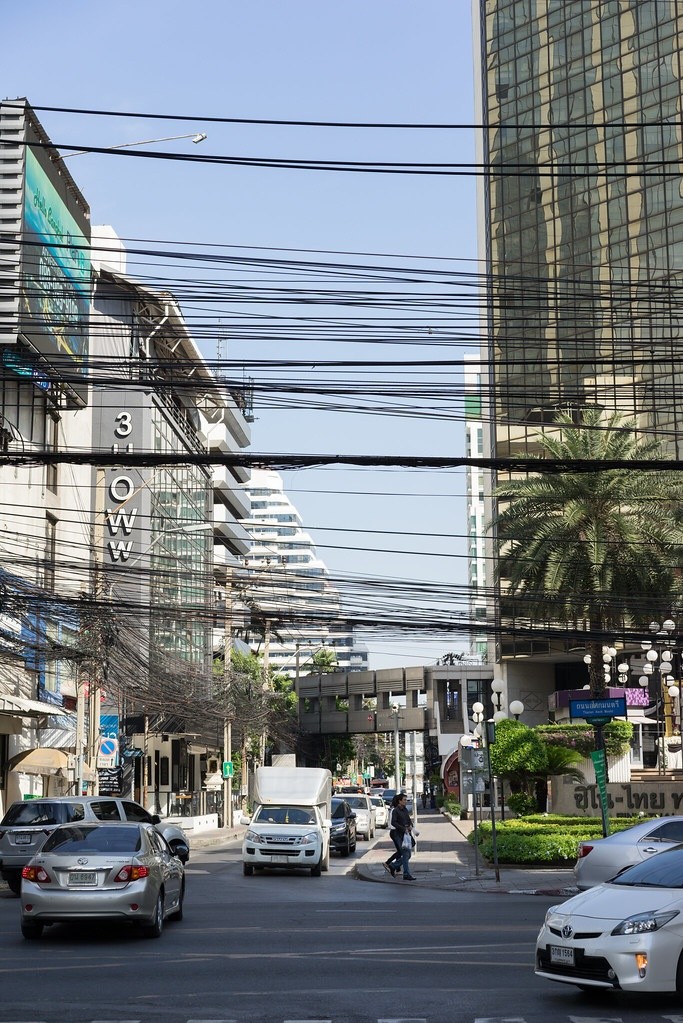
[383,794,416,880]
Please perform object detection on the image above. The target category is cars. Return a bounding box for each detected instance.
[573,814,683,893]
[382,789,397,809]
[21,819,188,940]
[333,778,390,829]
[533,843,683,1006]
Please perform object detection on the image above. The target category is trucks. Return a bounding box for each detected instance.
[240,766,333,878]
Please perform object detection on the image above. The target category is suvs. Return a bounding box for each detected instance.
[330,798,357,857]
[0,796,190,896]
[332,792,376,841]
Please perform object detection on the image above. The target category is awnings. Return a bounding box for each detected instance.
[0,694,71,718]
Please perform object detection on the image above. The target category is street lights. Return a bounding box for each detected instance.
[472,679,524,879]
[639,651,680,777]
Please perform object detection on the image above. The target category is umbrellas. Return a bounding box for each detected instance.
[6,747,96,797]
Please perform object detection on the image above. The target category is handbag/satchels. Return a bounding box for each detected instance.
[412,826,420,836]
[401,827,412,849]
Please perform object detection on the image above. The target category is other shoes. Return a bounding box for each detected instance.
[382,862,391,873]
[403,875,416,880]
[386,864,396,878]
[395,871,403,874]
